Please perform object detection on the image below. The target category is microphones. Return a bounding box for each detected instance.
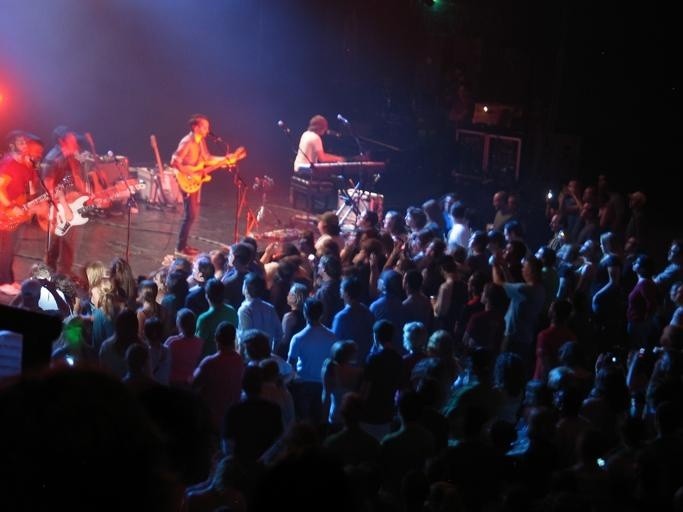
[107,150,125,168]
[337,113,350,124]
[277,120,296,136]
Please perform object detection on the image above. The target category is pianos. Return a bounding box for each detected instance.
[291,125,400,212]
[298,161,385,177]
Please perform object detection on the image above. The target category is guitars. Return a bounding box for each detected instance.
[2,175,75,232]
[175,146,247,193]
[39,180,147,236]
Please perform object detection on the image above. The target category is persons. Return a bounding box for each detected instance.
[293,116,347,181]
[40,126,113,285]
[0,130,44,295]
[0,164,683,511]
[170,112,237,256]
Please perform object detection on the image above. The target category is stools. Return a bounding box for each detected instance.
[289,176,334,211]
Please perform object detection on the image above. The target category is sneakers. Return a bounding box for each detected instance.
[175,248,197,257]
[11,282,23,290]
[0,284,21,294]
[186,245,199,252]
